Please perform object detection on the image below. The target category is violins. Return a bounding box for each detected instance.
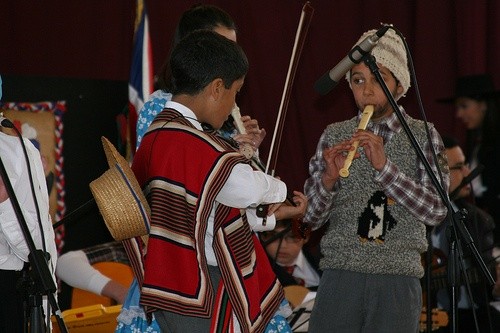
[219,129,309,240]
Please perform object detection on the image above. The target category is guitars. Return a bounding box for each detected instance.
[70,261,137,306]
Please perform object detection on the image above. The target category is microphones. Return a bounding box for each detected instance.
[314,26,390,95]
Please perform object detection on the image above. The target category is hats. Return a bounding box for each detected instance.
[435,75,500,107]
[88,136,151,246]
[346,27,411,100]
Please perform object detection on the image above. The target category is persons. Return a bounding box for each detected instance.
[259,200,320,288]
[115,5,293,333]
[130,29,287,333]
[420,72,500,333]
[301,24,450,333]
[0,126,131,333]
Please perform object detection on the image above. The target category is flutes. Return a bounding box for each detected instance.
[337,105,376,177]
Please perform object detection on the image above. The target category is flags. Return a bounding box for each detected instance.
[129,0,153,156]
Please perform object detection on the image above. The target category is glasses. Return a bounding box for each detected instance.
[449,164,465,172]
[258,229,302,244]
